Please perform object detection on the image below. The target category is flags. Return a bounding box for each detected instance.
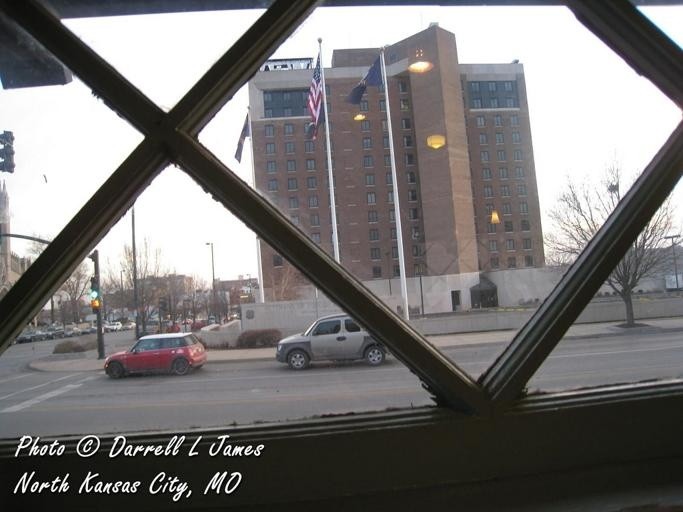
[304,47,324,143]
[232,110,251,164]
[341,51,385,106]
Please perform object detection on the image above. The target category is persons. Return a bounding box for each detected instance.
[153,321,168,332]
[167,321,180,346]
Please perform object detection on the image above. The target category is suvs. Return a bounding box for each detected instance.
[274,312,387,371]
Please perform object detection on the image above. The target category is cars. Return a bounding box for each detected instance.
[103,331,208,379]
[15,309,242,344]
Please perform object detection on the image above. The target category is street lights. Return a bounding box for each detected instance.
[206,242,220,324]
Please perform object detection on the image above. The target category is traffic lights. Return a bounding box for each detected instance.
[91,276,101,313]
